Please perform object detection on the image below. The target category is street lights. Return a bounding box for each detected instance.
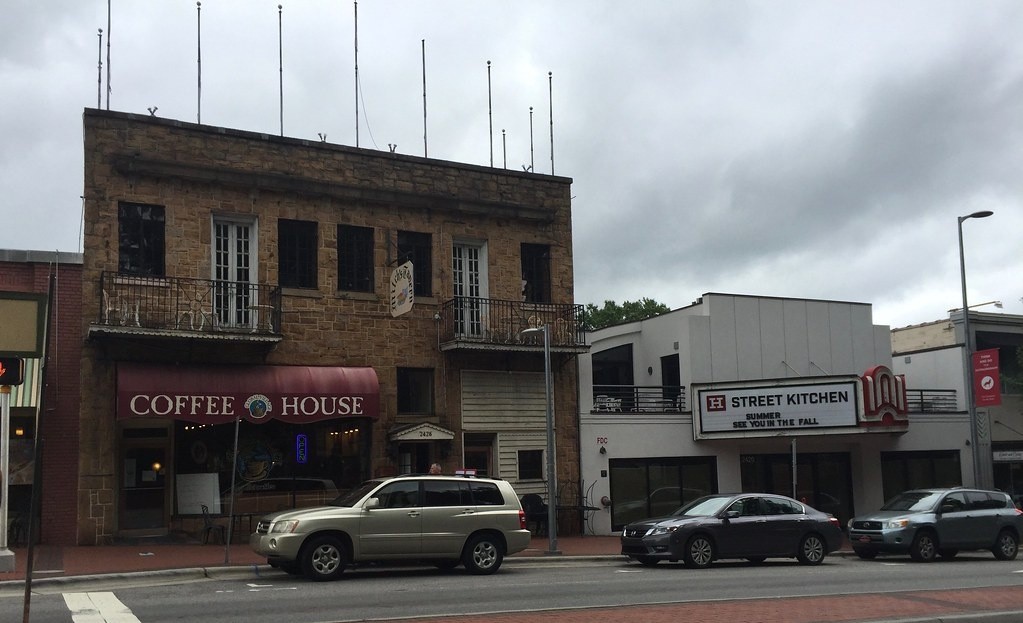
[520,321,561,554]
[956,209,997,496]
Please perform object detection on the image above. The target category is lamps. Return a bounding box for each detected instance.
[966,440,970,447]
[600,446,606,454]
[16,426,24,437]
[647,366,653,376]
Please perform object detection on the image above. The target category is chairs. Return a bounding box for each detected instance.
[7,498,38,548]
[174,292,225,332]
[631,394,687,413]
[198,504,225,545]
[590,394,624,415]
[527,316,574,343]
[102,289,128,325]
[520,493,554,533]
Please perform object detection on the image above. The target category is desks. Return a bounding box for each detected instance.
[246,303,274,333]
[500,315,523,344]
[229,511,264,541]
[555,506,602,537]
[118,292,151,327]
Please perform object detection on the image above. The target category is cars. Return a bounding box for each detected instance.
[618,493,846,566]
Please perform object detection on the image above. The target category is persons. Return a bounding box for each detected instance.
[429,463,443,475]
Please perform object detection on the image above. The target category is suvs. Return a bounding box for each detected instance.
[847,486,1023,561]
[247,474,530,574]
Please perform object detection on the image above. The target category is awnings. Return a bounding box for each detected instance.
[115,361,380,426]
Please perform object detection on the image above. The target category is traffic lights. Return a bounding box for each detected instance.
[0,357,25,387]
